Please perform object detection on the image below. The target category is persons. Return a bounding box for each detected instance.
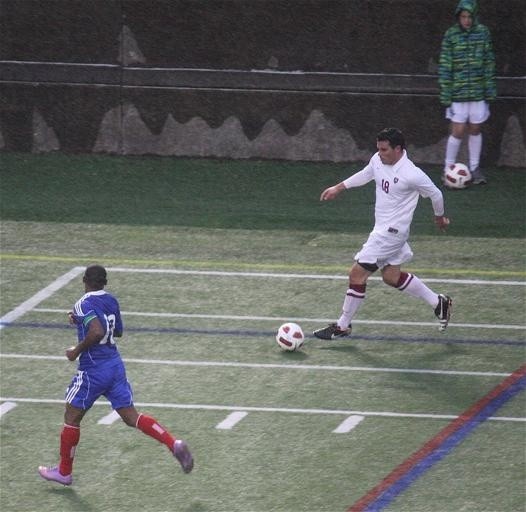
[312,127,452,339]
[38,264,193,484]
[438,0,496,185]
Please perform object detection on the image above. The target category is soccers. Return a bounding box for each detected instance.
[277,323,304,352]
[446,164,471,189]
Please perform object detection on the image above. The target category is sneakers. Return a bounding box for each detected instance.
[471,169,487,184]
[37,464,73,486]
[313,321,355,340]
[432,293,453,333]
[173,440,195,474]
